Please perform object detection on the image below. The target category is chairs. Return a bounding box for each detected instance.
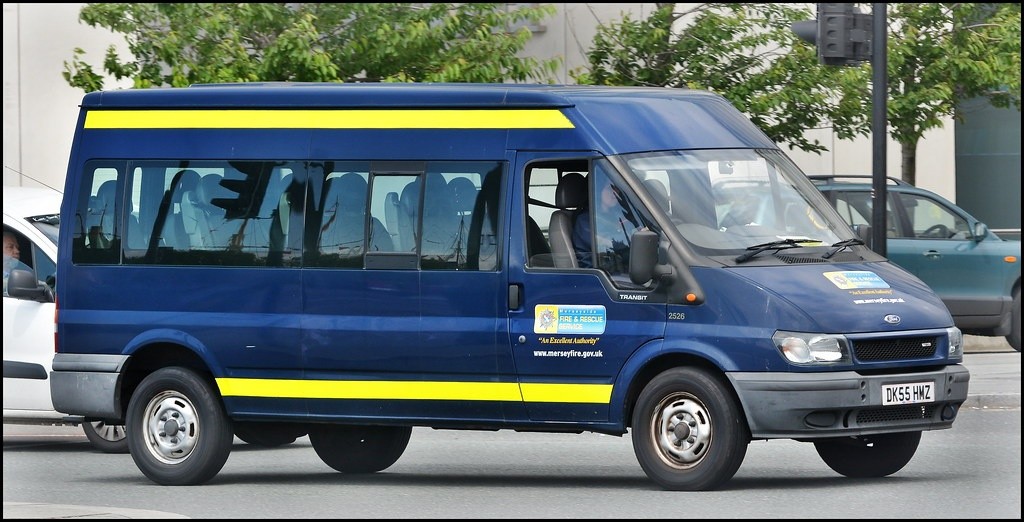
[90,168,680,291]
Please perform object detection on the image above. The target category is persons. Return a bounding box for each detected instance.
[3,230,20,297]
[571,171,650,269]
